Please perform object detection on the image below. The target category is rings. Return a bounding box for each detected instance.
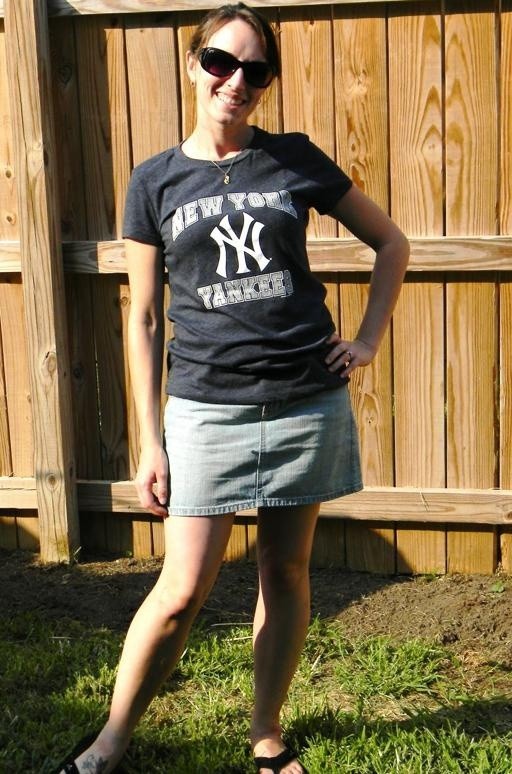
[346,352,352,358]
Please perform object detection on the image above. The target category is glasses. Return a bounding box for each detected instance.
[196,47,275,89]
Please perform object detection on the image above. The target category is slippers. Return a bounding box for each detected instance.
[47,731,99,774]
[254,746,308,774]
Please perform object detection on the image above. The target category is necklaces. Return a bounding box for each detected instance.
[210,153,239,185]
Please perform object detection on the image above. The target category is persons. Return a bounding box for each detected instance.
[51,4,411,774]
